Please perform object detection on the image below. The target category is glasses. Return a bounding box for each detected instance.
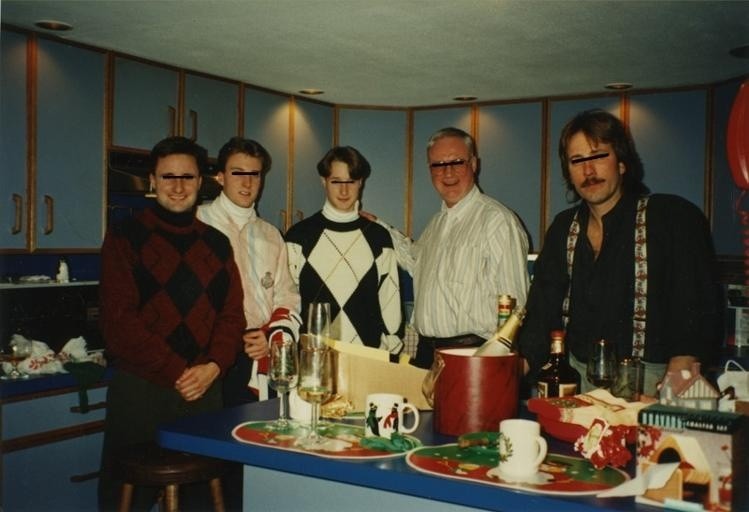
[427,159,466,176]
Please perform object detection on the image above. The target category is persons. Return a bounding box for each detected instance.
[193,138,304,402]
[512,109,731,399]
[354,126,535,372]
[282,146,403,366]
[97,136,246,512]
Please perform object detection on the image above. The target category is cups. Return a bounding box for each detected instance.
[363,394,420,440]
[499,418,548,479]
[611,356,642,402]
[587,337,617,390]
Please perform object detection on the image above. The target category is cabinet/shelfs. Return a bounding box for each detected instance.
[242,84,336,237]
[1,24,108,252]
[336,104,475,244]
[107,49,242,163]
[1,381,109,512]
[622,77,748,260]
[472,92,624,254]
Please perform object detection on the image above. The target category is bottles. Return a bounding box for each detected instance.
[537,330,579,398]
[497,295,513,333]
[474,306,528,357]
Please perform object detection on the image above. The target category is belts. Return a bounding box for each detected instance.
[419,335,487,349]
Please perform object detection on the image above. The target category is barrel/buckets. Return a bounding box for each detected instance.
[420,343,530,440]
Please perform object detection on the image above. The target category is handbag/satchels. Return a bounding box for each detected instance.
[717,359,748,401]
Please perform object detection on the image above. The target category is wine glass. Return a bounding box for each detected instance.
[306,302,331,431]
[299,349,332,448]
[266,341,300,432]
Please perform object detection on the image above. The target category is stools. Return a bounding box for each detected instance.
[112,440,238,512]
